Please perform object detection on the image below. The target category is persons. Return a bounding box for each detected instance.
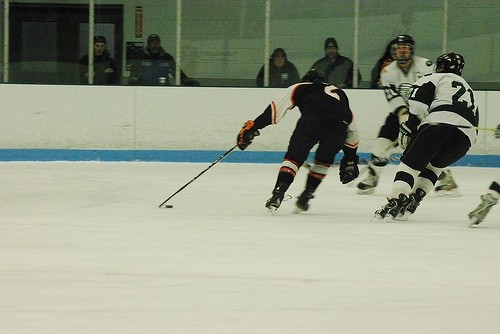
[356,34,461,199]
[468,172,500,224]
[308,37,364,89]
[256,48,301,89]
[238,67,360,216]
[374,52,481,221]
[371,37,399,88]
[131,34,191,85]
[78,36,122,85]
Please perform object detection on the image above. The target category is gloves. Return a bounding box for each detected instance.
[398,121,417,150]
[237,120,260,151]
[339,155,359,184]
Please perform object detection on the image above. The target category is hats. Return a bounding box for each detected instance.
[94,36,106,44]
[147,34,160,43]
[324,38,338,48]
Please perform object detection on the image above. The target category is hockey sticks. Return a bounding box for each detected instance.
[475,132,497,142]
[158,144,238,209]
[336,138,398,189]
[472,127,499,132]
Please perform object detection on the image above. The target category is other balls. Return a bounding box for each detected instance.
[164,205,173,208]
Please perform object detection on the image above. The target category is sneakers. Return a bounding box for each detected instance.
[371,193,408,223]
[296,191,314,211]
[394,188,426,220]
[468,194,497,227]
[265,191,292,215]
[434,170,460,196]
[357,167,378,195]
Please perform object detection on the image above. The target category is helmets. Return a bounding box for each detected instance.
[304,69,325,81]
[391,34,415,63]
[436,52,465,73]
